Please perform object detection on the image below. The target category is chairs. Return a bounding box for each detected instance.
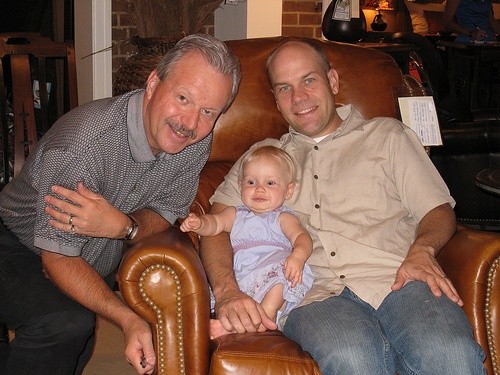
[0,31,78,192]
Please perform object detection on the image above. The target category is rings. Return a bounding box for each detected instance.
[70,224,76,234]
[69,215,73,224]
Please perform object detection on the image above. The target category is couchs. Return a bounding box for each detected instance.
[118,36,500,375]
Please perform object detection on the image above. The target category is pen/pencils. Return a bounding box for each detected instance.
[472,23,488,38]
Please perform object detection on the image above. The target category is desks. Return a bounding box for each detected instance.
[435,40,500,118]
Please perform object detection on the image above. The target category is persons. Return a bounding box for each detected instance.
[180,145,316,340]
[180,37,489,375]
[444,0,500,40]
[0,32,242,375]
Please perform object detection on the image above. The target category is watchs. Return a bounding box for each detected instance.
[126,213,139,240]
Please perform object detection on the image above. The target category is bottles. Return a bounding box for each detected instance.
[322,0,367,44]
[370,10,388,31]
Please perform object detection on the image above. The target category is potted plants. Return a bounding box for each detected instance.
[112,0,223,97]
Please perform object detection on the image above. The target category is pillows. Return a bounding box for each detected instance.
[404,0,447,38]
